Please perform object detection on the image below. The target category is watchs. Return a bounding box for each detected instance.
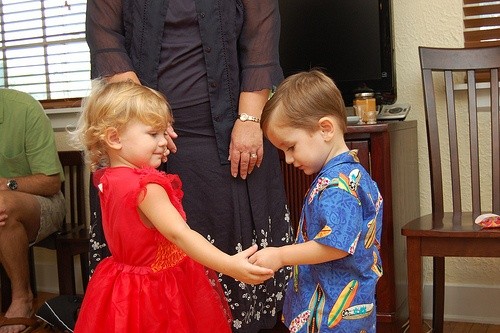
[6,177,19,191]
[236,113,261,123]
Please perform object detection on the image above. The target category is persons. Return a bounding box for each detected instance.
[249,71,383,333]
[0,87,65,333]
[85,0,294,333]
[64,79,274,333]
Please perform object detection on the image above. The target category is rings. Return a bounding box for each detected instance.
[250,153,258,158]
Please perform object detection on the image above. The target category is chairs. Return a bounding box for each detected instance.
[401,46,500,333]
[31,148,91,292]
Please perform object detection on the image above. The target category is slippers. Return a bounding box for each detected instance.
[0,315,42,333]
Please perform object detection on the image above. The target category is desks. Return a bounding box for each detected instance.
[279,120,420,333]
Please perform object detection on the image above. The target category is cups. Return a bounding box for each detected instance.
[352,86,383,125]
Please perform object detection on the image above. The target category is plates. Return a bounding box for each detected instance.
[347,117,361,126]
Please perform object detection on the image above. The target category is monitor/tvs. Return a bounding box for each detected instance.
[277,0,396,106]
